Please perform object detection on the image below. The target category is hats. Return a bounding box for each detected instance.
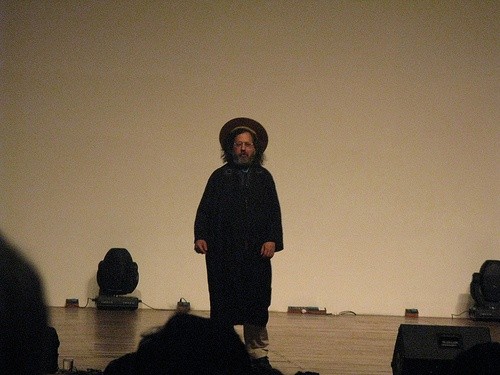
[219,118,268,154]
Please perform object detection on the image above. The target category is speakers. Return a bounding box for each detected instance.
[390,324,491,375]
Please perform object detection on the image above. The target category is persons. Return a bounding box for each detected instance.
[0,231,500,375]
[193,117,284,360]
